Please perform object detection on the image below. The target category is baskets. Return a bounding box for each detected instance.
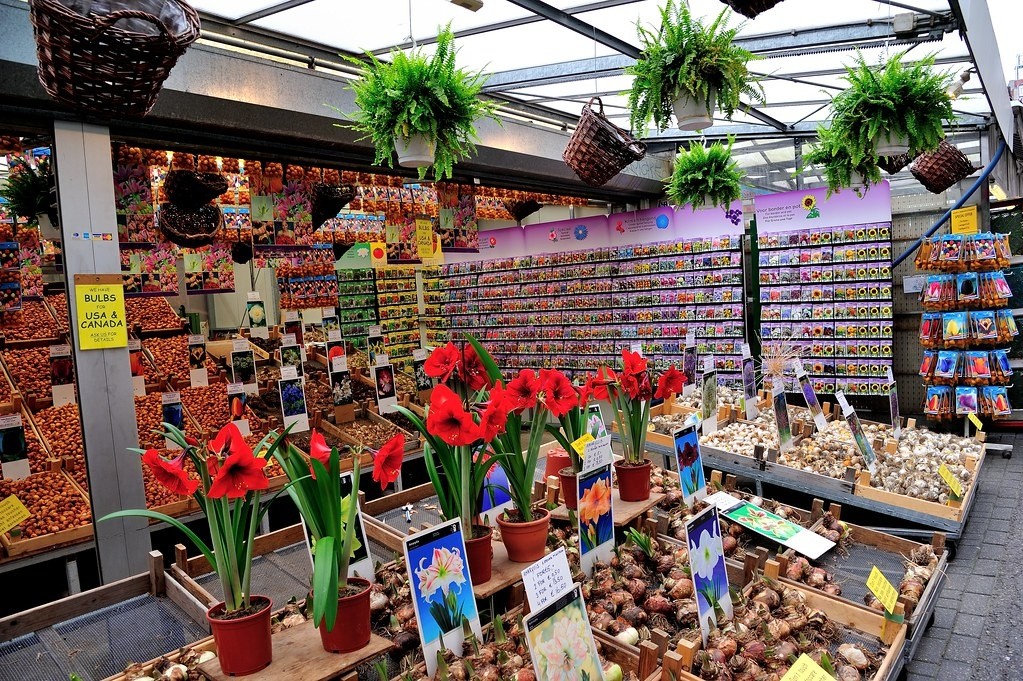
[162,161,228,212]
[561,96,648,189]
[311,182,356,219]
[910,140,985,195]
[876,134,947,175]
[158,203,222,248]
[29,0,202,120]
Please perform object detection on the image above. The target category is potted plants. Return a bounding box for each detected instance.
[661,136,757,215]
[323,22,507,180]
[627,9,767,139]
[797,47,955,197]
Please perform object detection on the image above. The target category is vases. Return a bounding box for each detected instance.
[206,459,652,674]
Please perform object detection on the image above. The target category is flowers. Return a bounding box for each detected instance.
[391,339,488,539]
[546,373,596,474]
[596,350,687,466]
[676,440,701,495]
[533,611,592,681]
[404,519,483,674]
[91,422,400,629]
[482,369,577,522]
[690,520,724,606]
[413,546,468,635]
[51,303,390,414]
[579,476,612,551]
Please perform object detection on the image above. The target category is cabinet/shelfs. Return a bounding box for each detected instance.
[757,224,894,397]
[422,235,747,387]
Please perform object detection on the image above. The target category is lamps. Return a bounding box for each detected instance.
[947,66,974,100]
